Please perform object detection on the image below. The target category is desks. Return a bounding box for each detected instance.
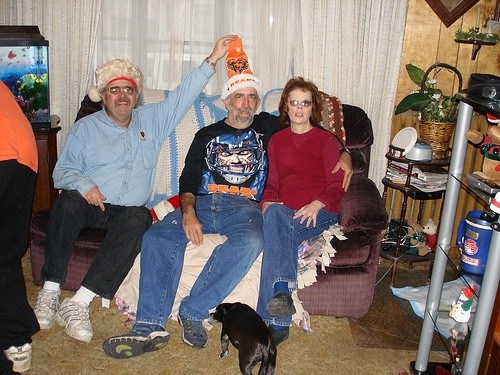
[27,115,62,220]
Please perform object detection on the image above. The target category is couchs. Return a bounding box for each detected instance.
[29,82,388,318]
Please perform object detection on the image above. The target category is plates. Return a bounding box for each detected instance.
[391,126,418,157]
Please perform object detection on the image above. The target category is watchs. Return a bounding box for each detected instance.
[340,148,351,154]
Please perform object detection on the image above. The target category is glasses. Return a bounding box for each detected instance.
[105,86,135,95]
[287,99,312,107]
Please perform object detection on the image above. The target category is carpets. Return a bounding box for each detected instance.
[345,266,455,351]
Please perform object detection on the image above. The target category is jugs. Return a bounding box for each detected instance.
[457,209,494,275]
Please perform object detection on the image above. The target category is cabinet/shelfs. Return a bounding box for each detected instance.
[382,144,452,286]
[411,86,500,375]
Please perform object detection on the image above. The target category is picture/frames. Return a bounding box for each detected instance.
[425,0,480,28]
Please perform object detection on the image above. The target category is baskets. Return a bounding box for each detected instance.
[417,63,463,160]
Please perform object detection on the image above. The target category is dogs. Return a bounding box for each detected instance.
[210,301,277,375]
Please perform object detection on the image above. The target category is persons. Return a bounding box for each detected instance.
[102,74,355,359]
[0,78,39,374]
[32,34,237,343]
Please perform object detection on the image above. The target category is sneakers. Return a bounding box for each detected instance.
[103,322,170,359]
[33,289,61,330]
[177,312,209,349]
[4,342,32,372]
[55,296,95,343]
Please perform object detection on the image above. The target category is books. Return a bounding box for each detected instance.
[386,161,447,192]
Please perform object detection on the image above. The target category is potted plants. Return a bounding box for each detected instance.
[394,62,465,154]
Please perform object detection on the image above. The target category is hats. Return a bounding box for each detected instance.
[220,34,262,99]
[88,59,144,102]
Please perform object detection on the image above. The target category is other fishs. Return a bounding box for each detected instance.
[0,46,45,66]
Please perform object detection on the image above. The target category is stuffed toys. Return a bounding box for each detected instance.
[410,219,438,255]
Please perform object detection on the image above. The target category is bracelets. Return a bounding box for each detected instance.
[206,57,216,67]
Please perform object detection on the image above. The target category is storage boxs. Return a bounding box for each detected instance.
[0,25,50,120]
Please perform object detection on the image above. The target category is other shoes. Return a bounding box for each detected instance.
[268,325,289,347]
[267,291,297,318]
